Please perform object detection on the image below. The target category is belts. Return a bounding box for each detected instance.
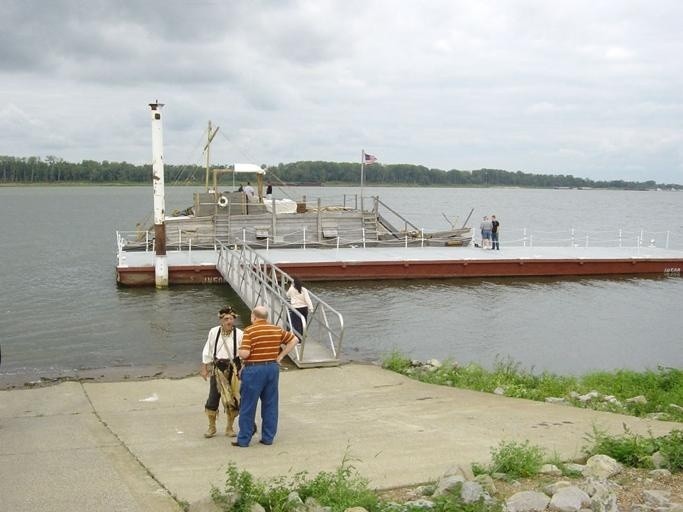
[246,361,276,366]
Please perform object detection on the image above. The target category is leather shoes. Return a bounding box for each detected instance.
[260,441,272,445]
[232,443,240,446]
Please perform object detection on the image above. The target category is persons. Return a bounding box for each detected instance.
[492,215,500,249]
[232,306,299,447]
[239,183,244,192]
[244,182,254,197]
[480,215,493,249]
[287,279,313,343]
[202,307,244,437]
[265,179,272,199]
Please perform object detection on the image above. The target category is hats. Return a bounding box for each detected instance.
[218,307,240,319]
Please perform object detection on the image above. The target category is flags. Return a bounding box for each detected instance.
[364,153,377,163]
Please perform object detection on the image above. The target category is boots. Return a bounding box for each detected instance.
[204,408,219,437]
[224,408,237,436]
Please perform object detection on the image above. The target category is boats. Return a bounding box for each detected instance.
[115,119,475,254]
